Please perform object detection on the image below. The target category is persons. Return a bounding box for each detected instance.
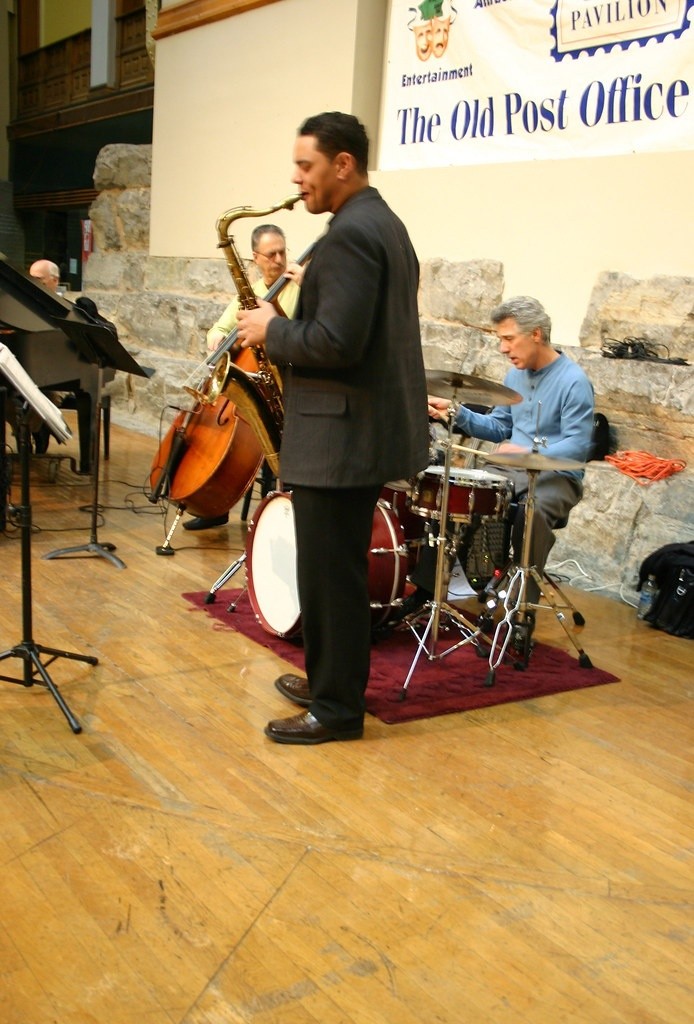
[16,259,60,454]
[206,225,299,351]
[403,296,594,644]
[236,112,429,743]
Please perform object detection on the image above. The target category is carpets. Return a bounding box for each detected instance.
[182,588,620,725]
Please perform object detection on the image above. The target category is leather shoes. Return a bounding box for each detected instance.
[274,674,313,704]
[264,709,364,744]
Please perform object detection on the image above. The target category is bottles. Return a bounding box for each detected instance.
[637,574,658,620]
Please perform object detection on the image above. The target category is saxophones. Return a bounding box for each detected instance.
[181,192,305,481]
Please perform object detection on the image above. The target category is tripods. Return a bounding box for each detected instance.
[0,315,155,734]
[397,388,594,702]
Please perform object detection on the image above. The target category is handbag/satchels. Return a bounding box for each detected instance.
[638,541,694,641]
[585,412,610,463]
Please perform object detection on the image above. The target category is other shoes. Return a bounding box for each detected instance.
[182,510,229,530]
[384,587,434,622]
[501,610,536,650]
[31,421,51,456]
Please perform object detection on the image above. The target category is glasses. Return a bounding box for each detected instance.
[254,248,288,260]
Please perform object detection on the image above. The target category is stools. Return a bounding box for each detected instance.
[478,515,585,634]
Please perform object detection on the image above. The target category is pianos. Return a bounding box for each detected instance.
[0,251,116,478]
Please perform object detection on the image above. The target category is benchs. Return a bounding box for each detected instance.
[60,395,110,461]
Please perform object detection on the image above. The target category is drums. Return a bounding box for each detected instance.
[411,464,515,526]
[379,479,415,536]
[244,490,409,642]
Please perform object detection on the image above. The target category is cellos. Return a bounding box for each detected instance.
[148,243,315,522]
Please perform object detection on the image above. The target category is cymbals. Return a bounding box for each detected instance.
[423,368,526,406]
[479,452,588,472]
